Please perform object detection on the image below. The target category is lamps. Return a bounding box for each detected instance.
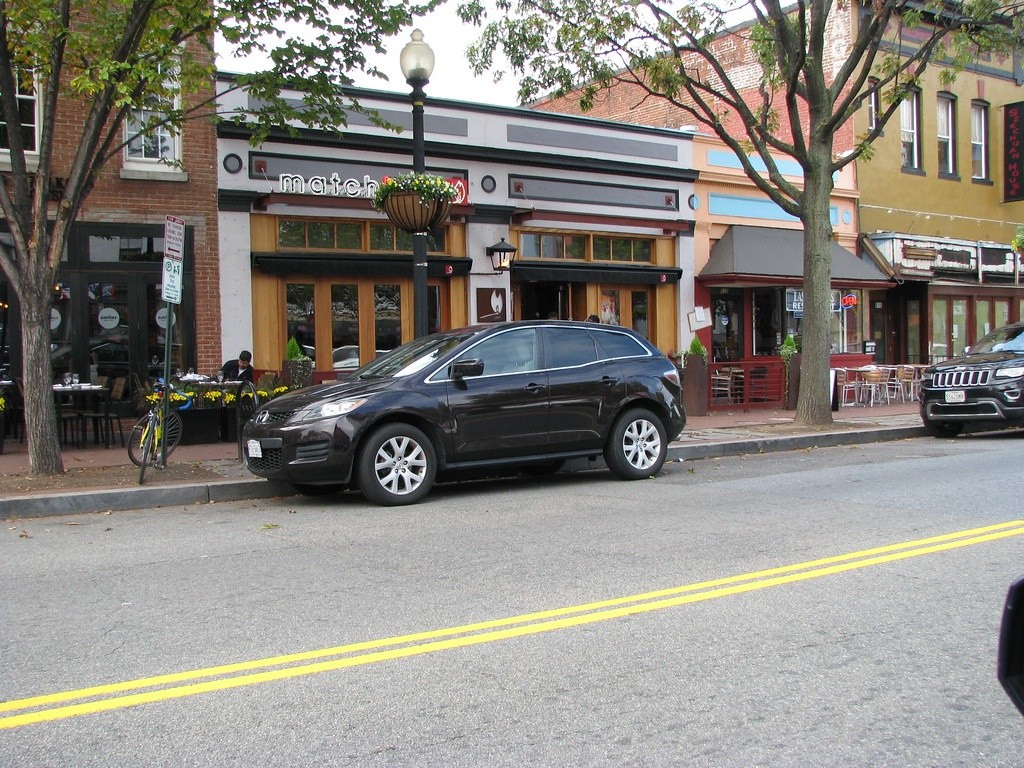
[489,238,519,274]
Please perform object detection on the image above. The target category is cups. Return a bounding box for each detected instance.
[72,374,79,383]
[176,369,182,378]
[188,368,194,375]
[64,373,72,383]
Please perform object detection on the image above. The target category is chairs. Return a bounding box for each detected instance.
[13,375,127,449]
[743,366,767,402]
[711,368,732,404]
[833,364,925,408]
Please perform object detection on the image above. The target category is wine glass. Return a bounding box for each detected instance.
[217,371,224,384]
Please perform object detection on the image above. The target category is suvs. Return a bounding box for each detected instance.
[918,321,1024,439]
[237,317,688,506]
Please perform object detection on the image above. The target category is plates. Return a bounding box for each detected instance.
[1,381,12,383]
[53,383,102,388]
[179,374,243,383]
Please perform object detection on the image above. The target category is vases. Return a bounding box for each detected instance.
[0,411,5,453]
[385,190,451,236]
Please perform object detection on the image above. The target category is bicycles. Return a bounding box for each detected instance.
[126,378,193,486]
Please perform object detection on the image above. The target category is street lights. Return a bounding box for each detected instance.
[374,27,453,339]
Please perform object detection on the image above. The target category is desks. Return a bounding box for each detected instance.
[843,367,873,408]
[912,364,930,402]
[177,380,244,444]
[720,369,754,406]
[881,366,899,402]
[52,384,110,450]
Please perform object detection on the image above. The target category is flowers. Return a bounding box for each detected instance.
[1011,227,1024,252]
[0,398,6,412]
[375,172,459,210]
[141,384,288,413]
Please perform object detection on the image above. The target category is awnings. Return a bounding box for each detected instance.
[699,226,897,288]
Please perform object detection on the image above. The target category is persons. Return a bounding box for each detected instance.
[548,313,558,320]
[216,350,253,386]
[588,315,599,323]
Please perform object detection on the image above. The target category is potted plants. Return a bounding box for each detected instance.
[682,335,709,417]
[282,337,313,392]
[780,336,804,410]
